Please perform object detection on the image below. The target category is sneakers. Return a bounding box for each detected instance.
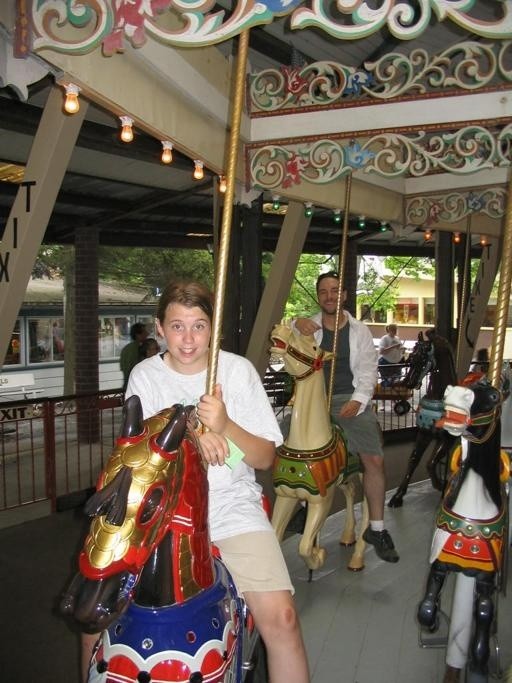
[361,523,400,563]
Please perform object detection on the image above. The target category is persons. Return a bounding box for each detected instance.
[424,326,455,397]
[305,266,400,567]
[118,321,149,389]
[124,279,312,682]
[377,323,407,414]
[136,337,160,364]
[46,321,61,354]
